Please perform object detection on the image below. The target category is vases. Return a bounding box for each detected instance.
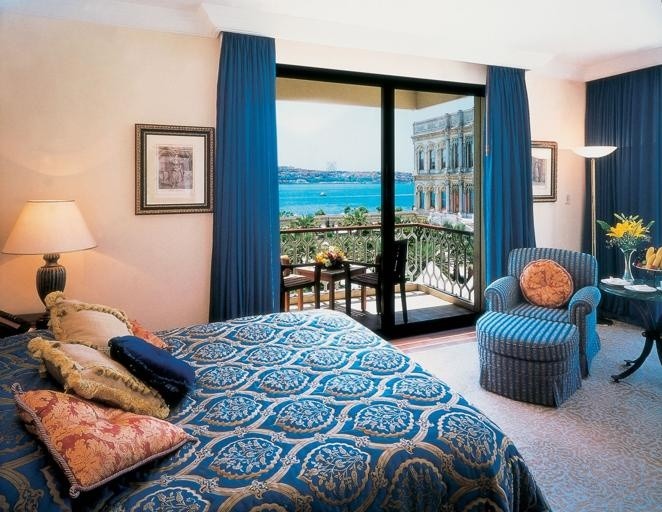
[325,260,343,270]
[620,247,638,285]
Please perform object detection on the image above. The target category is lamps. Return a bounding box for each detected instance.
[1,198,98,310]
[569,144,620,256]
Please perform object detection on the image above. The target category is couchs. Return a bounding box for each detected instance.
[475,246,602,409]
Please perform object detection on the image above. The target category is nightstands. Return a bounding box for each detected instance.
[0,311,48,339]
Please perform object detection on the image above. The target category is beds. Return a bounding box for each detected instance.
[0,306,556,512]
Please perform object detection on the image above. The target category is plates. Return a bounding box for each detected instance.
[601,279,630,287]
[624,285,657,292]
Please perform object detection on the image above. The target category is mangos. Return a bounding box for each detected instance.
[657,247,662,258]
[652,254,661,269]
[646,247,654,263]
[647,254,656,268]
[660,259,662,269]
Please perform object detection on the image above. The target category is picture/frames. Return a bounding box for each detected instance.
[133,122,216,216]
[530,138,559,205]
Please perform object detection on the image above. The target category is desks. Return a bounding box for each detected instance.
[294,263,368,311]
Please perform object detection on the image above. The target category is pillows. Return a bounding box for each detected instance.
[9,381,191,498]
[25,336,172,421]
[45,291,135,359]
[519,257,574,310]
[126,319,173,356]
[106,335,197,399]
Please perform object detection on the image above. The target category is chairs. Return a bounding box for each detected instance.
[280,254,323,313]
[343,238,409,322]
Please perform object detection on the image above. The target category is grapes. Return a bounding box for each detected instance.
[637,246,647,262]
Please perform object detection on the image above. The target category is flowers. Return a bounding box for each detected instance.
[596,212,658,278]
[315,245,348,268]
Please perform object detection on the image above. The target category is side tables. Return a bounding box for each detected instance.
[598,277,662,384]
